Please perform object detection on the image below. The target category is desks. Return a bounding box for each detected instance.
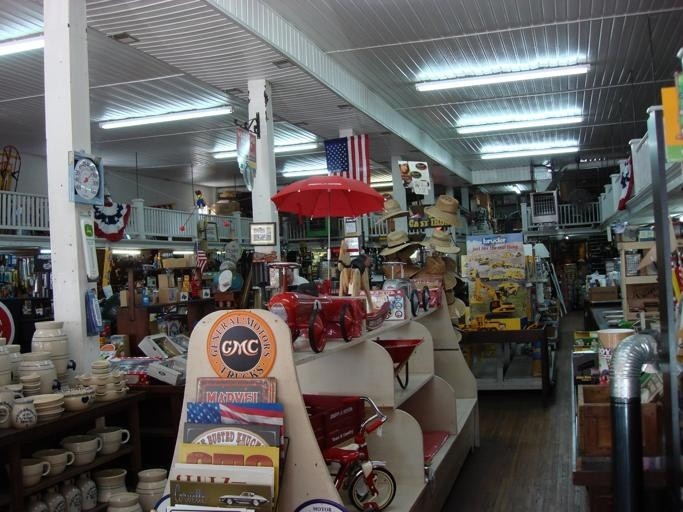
[460,321,561,409]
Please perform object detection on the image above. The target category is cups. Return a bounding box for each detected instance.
[89,428,130,454]
[32,450,75,476]
[21,459,50,487]
[61,435,103,466]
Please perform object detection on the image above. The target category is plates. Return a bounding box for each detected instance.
[27,393,65,421]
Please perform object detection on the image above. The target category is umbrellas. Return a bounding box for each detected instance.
[270,175,387,294]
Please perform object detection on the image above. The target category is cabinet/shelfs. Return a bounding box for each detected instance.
[111,263,253,357]
[128,381,185,472]
[169,286,483,510]
[586,162,682,448]
[0,387,151,511]
[535,279,561,341]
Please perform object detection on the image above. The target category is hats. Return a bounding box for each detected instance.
[381,258,422,278]
[374,199,409,224]
[444,289,466,320]
[378,229,421,257]
[441,256,462,280]
[418,230,461,254]
[412,256,457,291]
[423,195,465,226]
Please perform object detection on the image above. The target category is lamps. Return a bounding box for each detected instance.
[415,62,591,93]
[210,142,319,160]
[456,114,585,136]
[98,105,236,130]
[480,145,580,161]
[0,32,44,57]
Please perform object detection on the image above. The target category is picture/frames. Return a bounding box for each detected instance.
[249,222,277,246]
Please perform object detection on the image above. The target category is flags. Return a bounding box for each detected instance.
[193,239,208,273]
[324,133,370,187]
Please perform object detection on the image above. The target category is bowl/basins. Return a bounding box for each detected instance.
[56,384,96,412]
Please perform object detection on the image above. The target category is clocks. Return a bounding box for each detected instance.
[69,151,99,204]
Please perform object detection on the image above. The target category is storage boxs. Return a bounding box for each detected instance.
[577,383,657,466]
